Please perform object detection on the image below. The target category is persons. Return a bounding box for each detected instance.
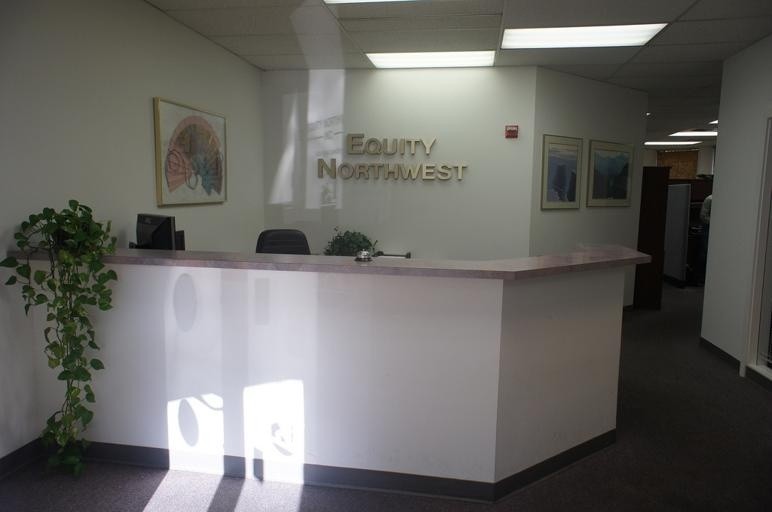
[699,194,713,225]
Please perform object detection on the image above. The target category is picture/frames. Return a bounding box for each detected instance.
[586,137,636,210]
[538,131,585,211]
[152,93,230,209]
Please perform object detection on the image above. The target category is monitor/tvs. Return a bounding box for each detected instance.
[137,213,176,250]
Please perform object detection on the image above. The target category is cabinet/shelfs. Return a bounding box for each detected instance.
[632,163,673,313]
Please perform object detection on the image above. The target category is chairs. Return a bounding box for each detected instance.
[256,225,311,255]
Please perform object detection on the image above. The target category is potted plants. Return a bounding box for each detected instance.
[2,196,124,480]
